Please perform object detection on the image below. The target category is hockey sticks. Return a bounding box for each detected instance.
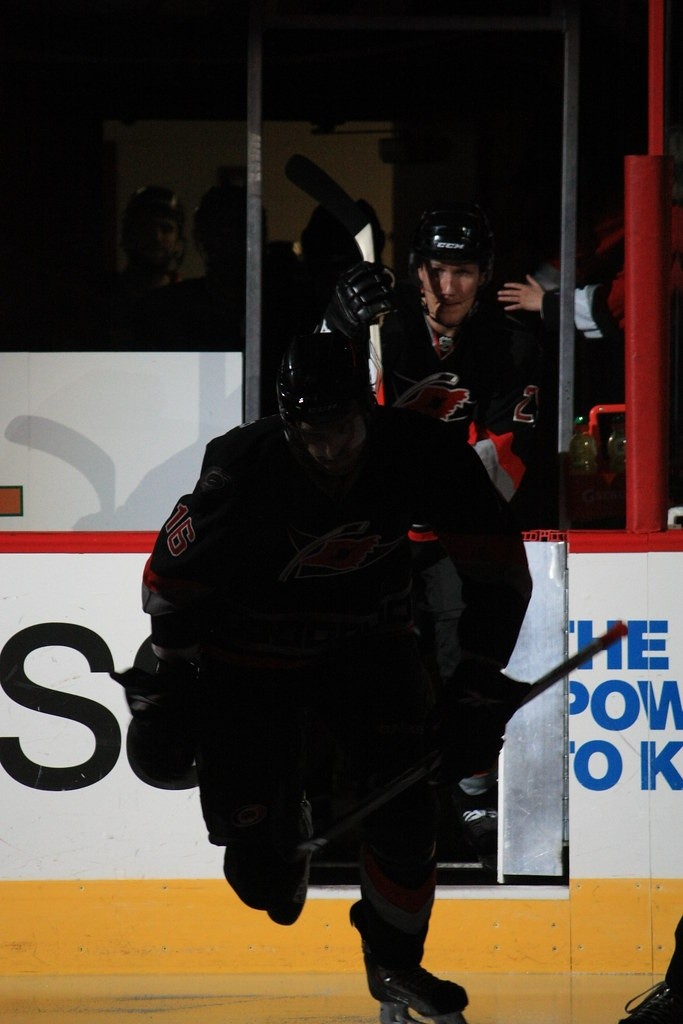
[258,614,634,865]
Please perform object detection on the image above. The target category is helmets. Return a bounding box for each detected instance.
[409,202,496,266]
[277,333,371,427]
[122,185,185,241]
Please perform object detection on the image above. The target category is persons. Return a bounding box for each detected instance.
[618,914,683,1024]
[146,334,531,1024]
[82,146,683,824]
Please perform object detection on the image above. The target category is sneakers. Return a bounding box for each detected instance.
[266,800,313,925]
[350,900,470,1024]
[618,982,683,1024]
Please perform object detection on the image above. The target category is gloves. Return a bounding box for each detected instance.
[319,261,395,346]
[424,663,503,773]
